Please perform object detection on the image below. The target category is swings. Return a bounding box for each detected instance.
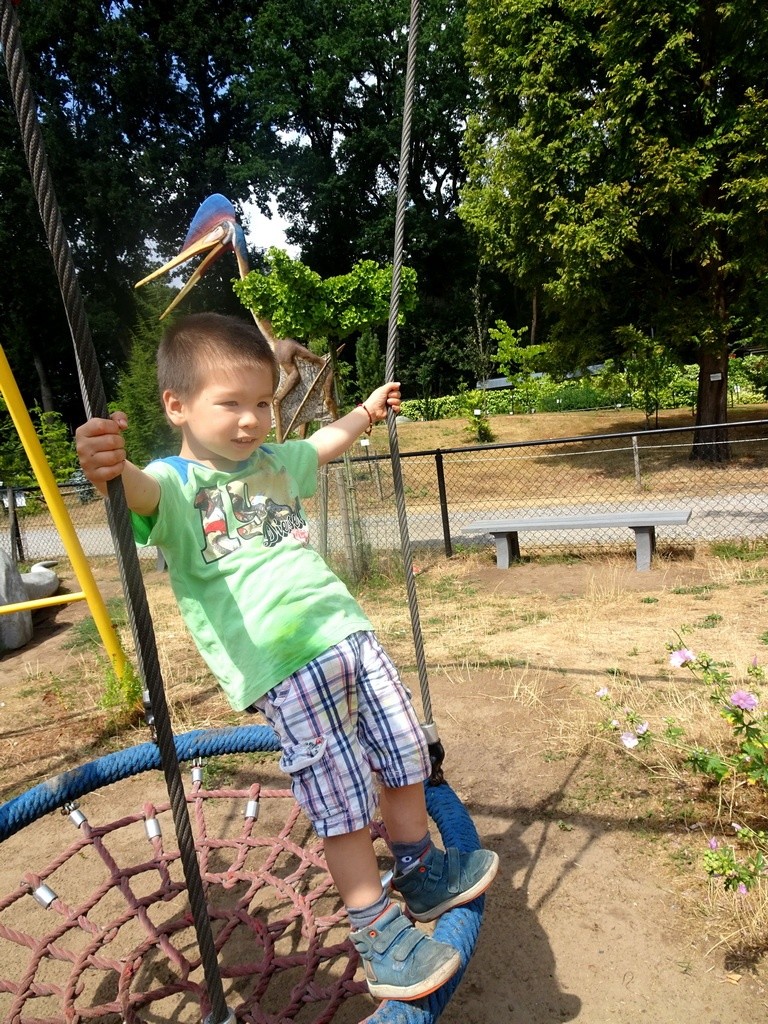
[1,2,489,1024]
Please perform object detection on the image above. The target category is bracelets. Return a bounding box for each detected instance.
[357,401,374,436]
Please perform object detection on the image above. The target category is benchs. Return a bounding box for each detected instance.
[461,510,691,569]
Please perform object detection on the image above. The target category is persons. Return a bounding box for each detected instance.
[71,310,502,1008]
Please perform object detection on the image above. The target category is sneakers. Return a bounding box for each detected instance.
[391,840,500,923]
[348,903,461,1001]
